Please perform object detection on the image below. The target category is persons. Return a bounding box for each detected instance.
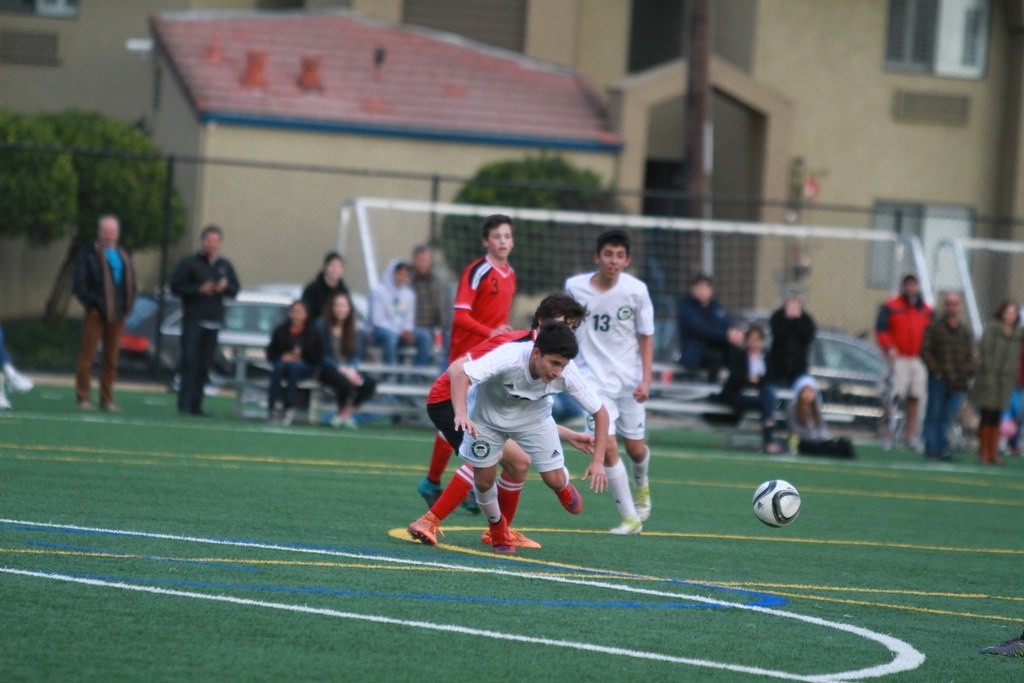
[877,275,937,454]
[730,325,785,453]
[920,292,981,463]
[0,328,33,409]
[301,253,348,319]
[450,322,610,553]
[564,230,655,534]
[416,215,516,516]
[766,295,818,389]
[972,301,1024,464]
[371,245,445,384]
[315,293,375,430]
[169,226,242,416]
[789,377,829,440]
[679,275,744,383]
[407,293,596,547]
[267,300,322,426]
[73,214,137,414]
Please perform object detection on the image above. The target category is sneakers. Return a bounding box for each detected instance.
[481,527,541,548]
[459,490,481,515]
[634,486,650,521]
[418,476,442,510]
[556,483,582,514]
[979,635,1024,659]
[610,518,643,534]
[407,515,446,545]
[489,515,515,554]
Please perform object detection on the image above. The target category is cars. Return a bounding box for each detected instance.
[94,283,444,395]
[640,311,961,459]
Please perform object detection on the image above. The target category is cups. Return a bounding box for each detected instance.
[662,369,673,384]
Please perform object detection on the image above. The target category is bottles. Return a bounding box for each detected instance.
[788,431,800,456]
[434,327,443,352]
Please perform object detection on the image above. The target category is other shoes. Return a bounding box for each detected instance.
[5,375,35,395]
[331,414,357,431]
[77,400,93,412]
[102,403,122,415]
[905,434,925,454]
[764,442,782,454]
[929,453,961,464]
[879,435,895,453]
[762,418,775,429]
[268,407,294,428]
[0,396,12,411]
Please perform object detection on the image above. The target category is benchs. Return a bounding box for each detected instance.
[217,330,803,460]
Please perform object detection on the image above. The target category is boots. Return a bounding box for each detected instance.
[991,428,1004,465]
[979,426,991,465]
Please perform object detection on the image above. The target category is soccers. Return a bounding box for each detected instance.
[752,480,801,528]
